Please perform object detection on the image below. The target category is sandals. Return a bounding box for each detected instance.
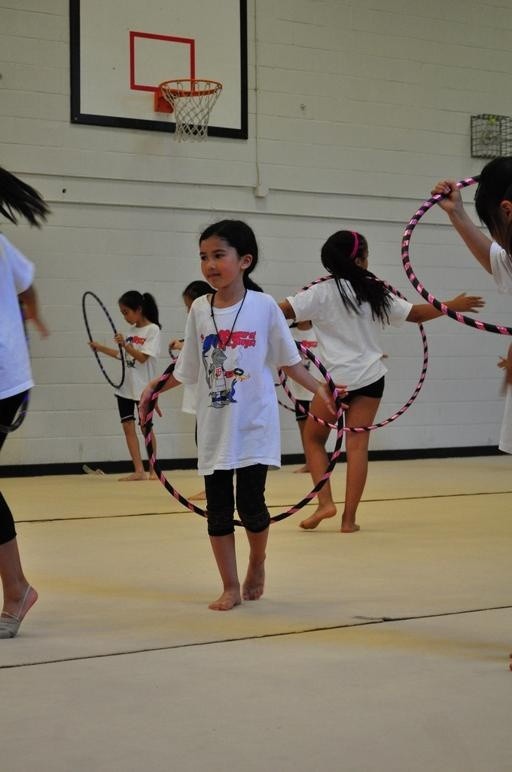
[0,584,32,639]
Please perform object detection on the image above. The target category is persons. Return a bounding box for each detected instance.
[0,165,52,640]
[430,157,512,455]
